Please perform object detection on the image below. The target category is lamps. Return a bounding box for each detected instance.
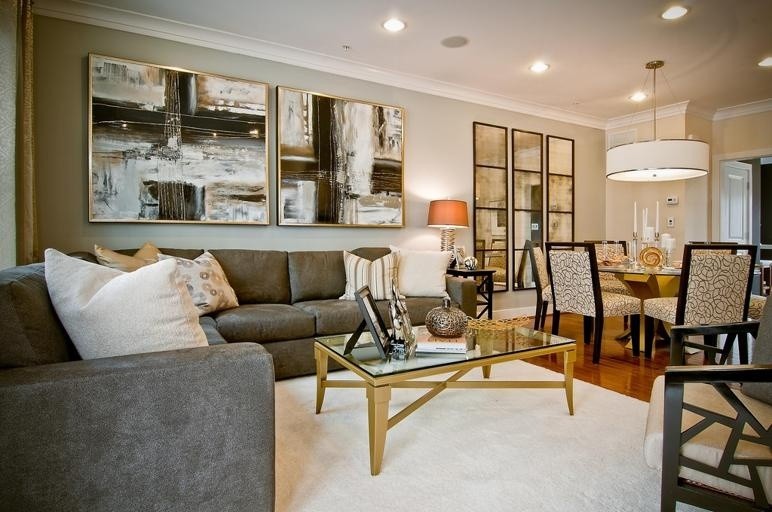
[606,59,710,183]
[426,198,469,265]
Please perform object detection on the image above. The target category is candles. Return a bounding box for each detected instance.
[656,201,659,233]
[633,201,638,232]
[643,208,648,240]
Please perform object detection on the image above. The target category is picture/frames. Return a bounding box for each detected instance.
[87,52,271,227]
[340,286,390,362]
[274,85,405,228]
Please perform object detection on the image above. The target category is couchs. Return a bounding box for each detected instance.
[1,247,478,512]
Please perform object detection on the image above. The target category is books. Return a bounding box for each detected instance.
[414,327,468,354]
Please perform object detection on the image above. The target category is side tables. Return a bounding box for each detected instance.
[446,267,497,318]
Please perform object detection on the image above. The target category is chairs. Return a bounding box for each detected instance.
[644,286,772,512]
[528,240,772,363]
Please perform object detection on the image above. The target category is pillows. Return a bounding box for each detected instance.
[92,244,155,273]
[44,248,209,360]
[157,247,240,317]
[339,249,405,302]
[135,241,162,262]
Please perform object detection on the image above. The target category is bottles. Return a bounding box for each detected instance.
[464,256,479,270]
[601,240,637,270]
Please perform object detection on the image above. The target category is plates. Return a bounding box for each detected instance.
[639,246,664,270]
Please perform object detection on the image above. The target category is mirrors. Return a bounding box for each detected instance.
[475,121,574,290]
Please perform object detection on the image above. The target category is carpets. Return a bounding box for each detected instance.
[276,346,706,512]
